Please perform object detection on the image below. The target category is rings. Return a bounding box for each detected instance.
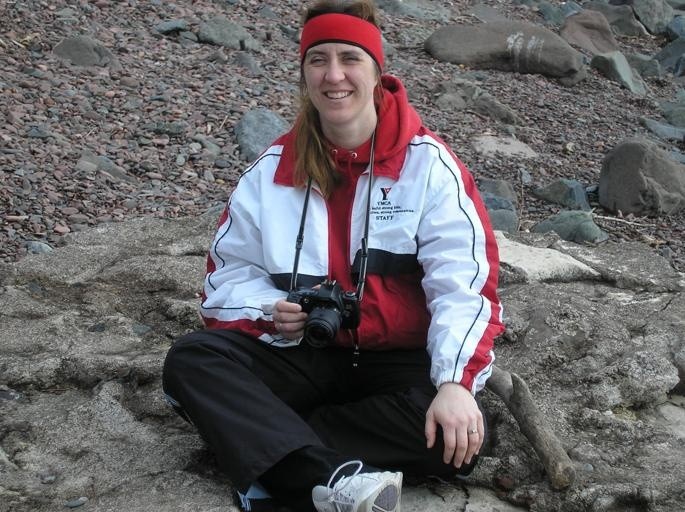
[279,323,281,331]
[468,430,478,434]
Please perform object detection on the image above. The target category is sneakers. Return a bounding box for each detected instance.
[310,466,405,511]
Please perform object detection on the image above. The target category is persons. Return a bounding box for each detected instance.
[162,1,506,512]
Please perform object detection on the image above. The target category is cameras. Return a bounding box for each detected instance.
[287,279,360,349]
